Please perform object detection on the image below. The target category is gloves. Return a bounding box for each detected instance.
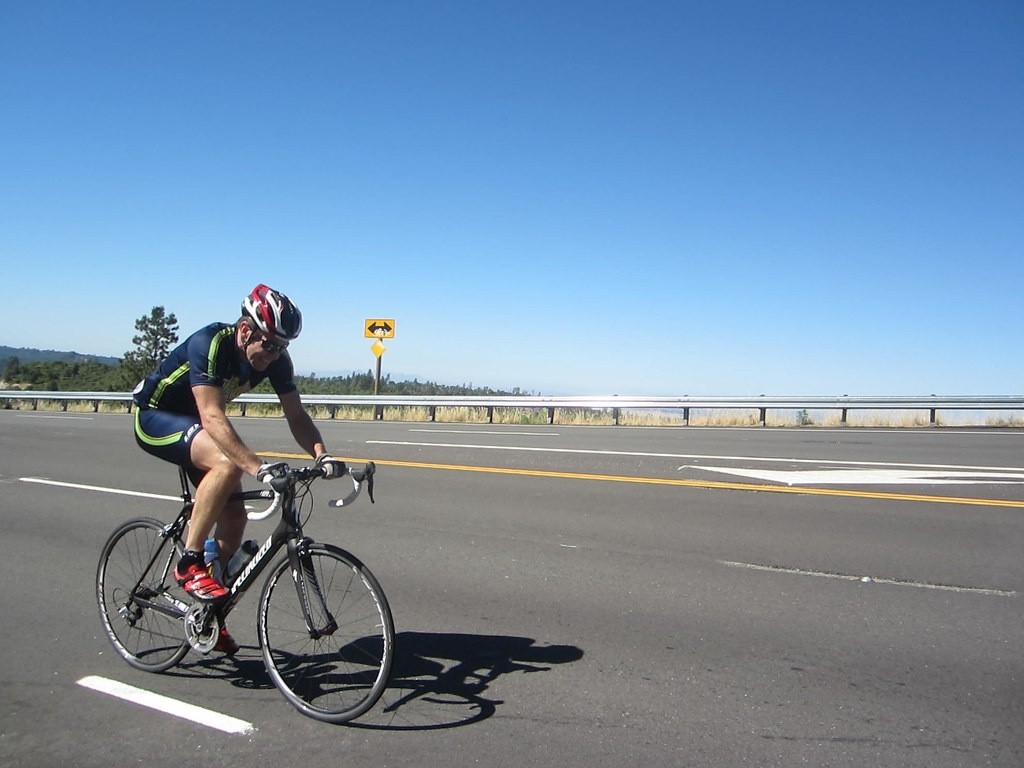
[314,454,346,481]
[255,460,297,494]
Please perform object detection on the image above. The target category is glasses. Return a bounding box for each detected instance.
[260,338,290,354]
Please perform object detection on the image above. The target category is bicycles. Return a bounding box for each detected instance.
[94,458,396,725]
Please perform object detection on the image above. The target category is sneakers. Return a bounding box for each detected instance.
[173,562,233,604]
[209,625,241,654]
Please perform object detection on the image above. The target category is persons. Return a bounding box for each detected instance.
[130,284,347,652]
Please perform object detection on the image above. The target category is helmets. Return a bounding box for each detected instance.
[240,284,302,341]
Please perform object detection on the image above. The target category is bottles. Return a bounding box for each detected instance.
[202,537,221,583]
[221,539,260,590]
